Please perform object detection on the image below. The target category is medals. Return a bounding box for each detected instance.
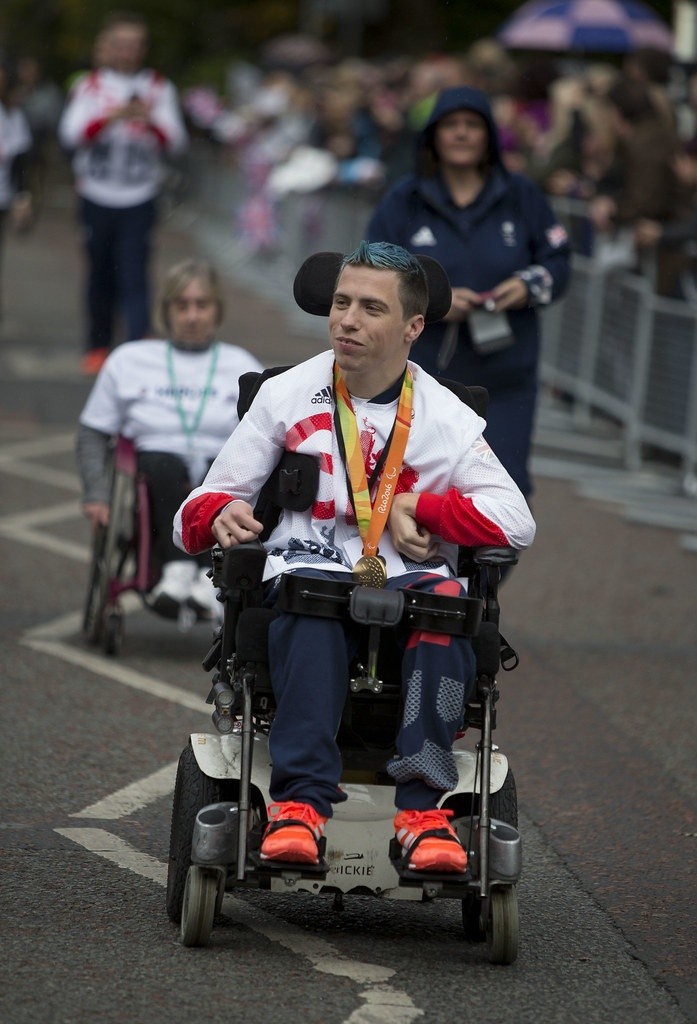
[353,556,387,590]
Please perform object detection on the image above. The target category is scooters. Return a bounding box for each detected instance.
[168,249,522,966]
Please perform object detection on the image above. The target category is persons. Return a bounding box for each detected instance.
[173,242,536,875]
[1,15,192,370]
[211,40,696,466]
[74,257,264,621]
[364,87,573,520]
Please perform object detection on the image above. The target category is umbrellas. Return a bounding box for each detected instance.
[501,0,676,53]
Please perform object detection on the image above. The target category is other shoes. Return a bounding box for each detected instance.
[149,559,224,623]
[84,349,110,375]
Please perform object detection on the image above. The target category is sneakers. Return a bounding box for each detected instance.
[394,805,468,874]
[261,800,328,865]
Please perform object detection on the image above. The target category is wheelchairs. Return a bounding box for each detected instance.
[83,433,224,657]
